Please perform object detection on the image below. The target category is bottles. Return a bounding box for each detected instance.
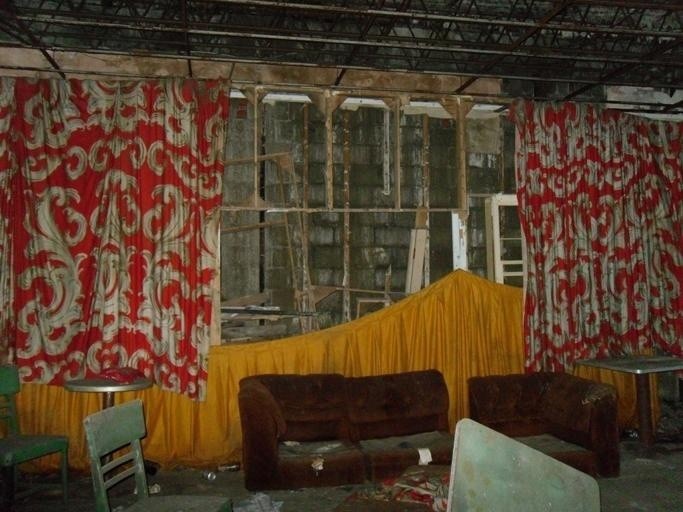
[217,462,244,472]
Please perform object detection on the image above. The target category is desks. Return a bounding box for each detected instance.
[65,376,154,499]
[577,357,682,458]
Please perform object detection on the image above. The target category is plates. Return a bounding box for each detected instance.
[639,353,677,362]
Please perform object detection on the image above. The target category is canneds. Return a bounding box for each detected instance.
[200,469,216,480]
[218,463,239,472]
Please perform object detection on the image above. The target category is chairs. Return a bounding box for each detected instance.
[82,399,233,511]
[1,365,72,510]
